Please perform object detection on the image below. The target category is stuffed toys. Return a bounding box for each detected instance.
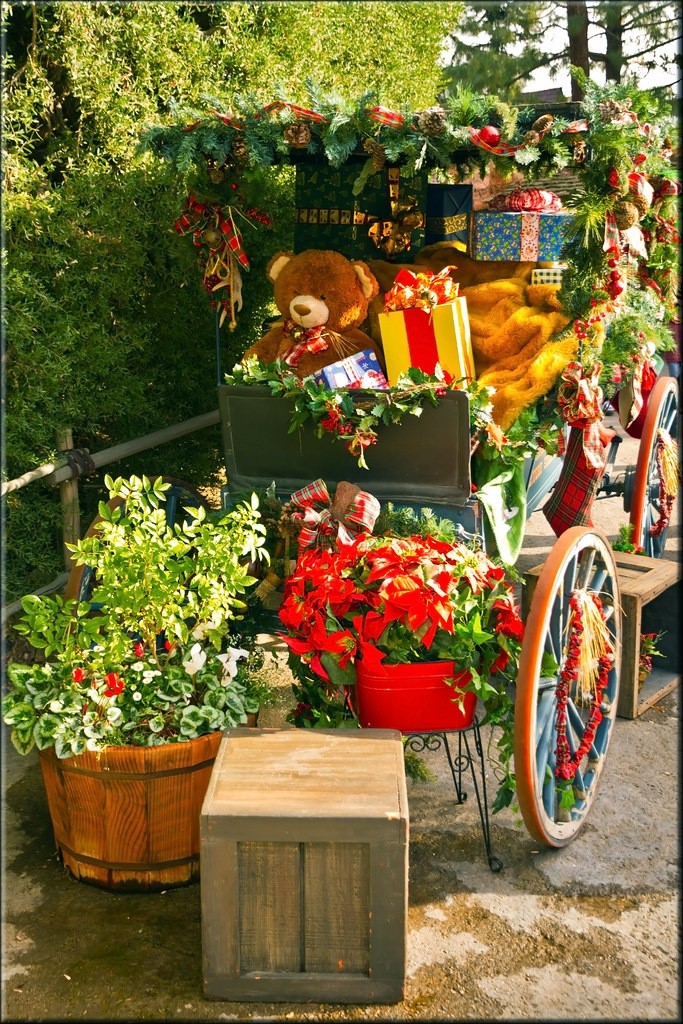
[241,250,387,382]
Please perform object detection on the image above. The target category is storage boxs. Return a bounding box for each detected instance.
[473,209,577,262]
[522,550,683,719]
[424,183,472,245]
[377,295,477,392]
[199,727,410,1005]
[309,349,391,390]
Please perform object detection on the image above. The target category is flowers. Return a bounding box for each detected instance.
[1,475,273,760]
[275,530,578,829]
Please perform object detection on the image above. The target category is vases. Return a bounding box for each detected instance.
[352,658,477,734]
[36,727,225,893]
